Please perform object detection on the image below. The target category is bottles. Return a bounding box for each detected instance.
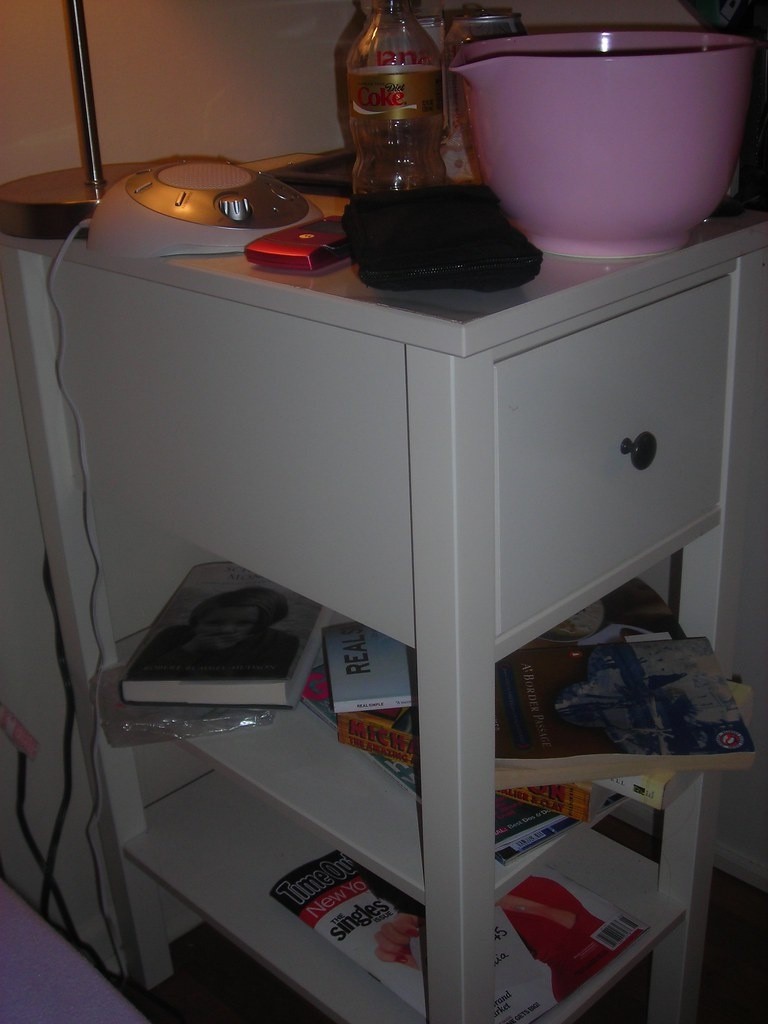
[344,0,443,196]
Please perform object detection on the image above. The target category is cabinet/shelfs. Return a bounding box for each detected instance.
[0,149,768,1024]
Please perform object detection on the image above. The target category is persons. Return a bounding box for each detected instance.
[375,874,643,1003]
[127,586,298,680]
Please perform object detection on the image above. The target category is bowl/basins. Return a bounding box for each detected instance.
[446,28,756,259]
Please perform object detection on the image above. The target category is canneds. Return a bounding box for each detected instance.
[356,3,529,186]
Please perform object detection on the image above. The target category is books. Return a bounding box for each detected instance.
[268,849,650,1024]
[119,562,335,711]
[300,621,757,867]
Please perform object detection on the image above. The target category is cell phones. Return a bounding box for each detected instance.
[244,216,349,271]
